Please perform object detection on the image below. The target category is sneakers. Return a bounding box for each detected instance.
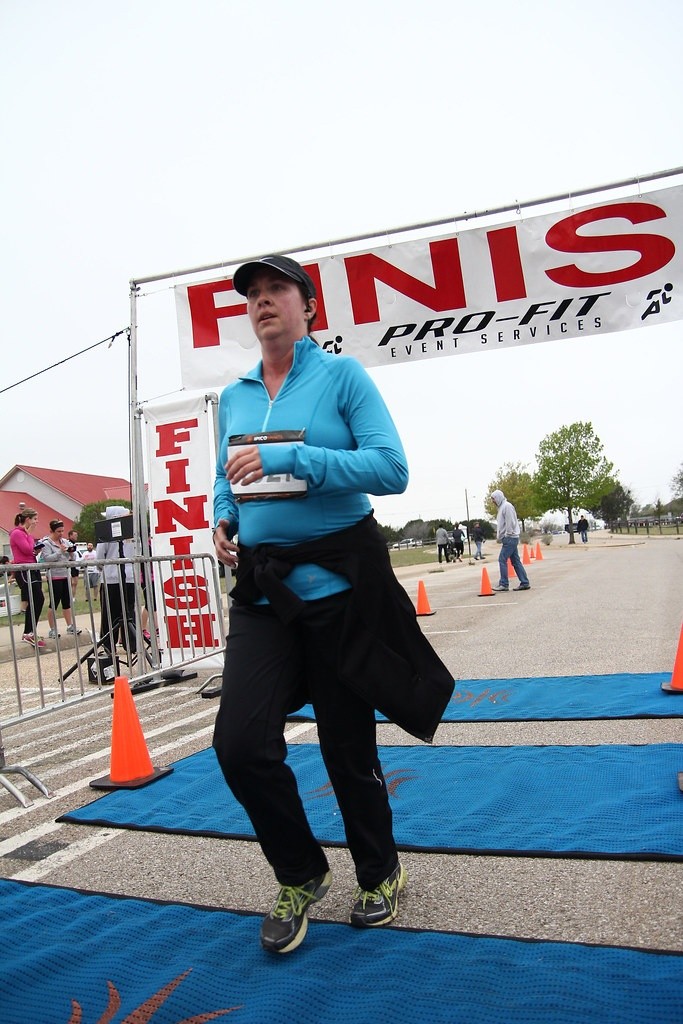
[513,583,531,591]
[143,630,150,638]
[22,632,46,647]
[347,861,401,925]
[67,626,82,636]
[49,630,61,639]
[260,868,332,955]
[492,584,509,591]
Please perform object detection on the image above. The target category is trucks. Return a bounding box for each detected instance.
[565,523,578,533]
[74,543,87,569]
[393,538,423,549]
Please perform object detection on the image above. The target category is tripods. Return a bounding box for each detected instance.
[58,539,159,682]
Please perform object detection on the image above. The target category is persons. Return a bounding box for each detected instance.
[96,505,142,657]
[211,254,409,955]
[577,515,589,544]
[81,541,98,601]
[0,555,16,585]
[9,508,46,648]
[42,519,82,638]
[472,522,486,560]
[491,490,530,591]
[453,522,465,562]
[435,524,452,563]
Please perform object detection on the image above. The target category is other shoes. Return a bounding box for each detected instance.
[481,556,485,559]
[474,558,479,560]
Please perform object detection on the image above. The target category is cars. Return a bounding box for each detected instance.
[592,525,601,530]
[558,531,567,534]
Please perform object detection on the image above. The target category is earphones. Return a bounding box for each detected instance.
[304,306,311,313]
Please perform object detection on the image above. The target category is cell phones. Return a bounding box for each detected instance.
[33,543,45,550]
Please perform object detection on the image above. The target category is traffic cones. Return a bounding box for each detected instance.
[522,544,532,564]
[507,558,517,577]
[89,676,174,791]
[477,567,497,597]
[416,581,436,616]
[536,543,543,560]
[530,547,536,558]
[661,623,683,694]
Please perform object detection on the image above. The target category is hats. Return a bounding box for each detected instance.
[234,257,314,320]
